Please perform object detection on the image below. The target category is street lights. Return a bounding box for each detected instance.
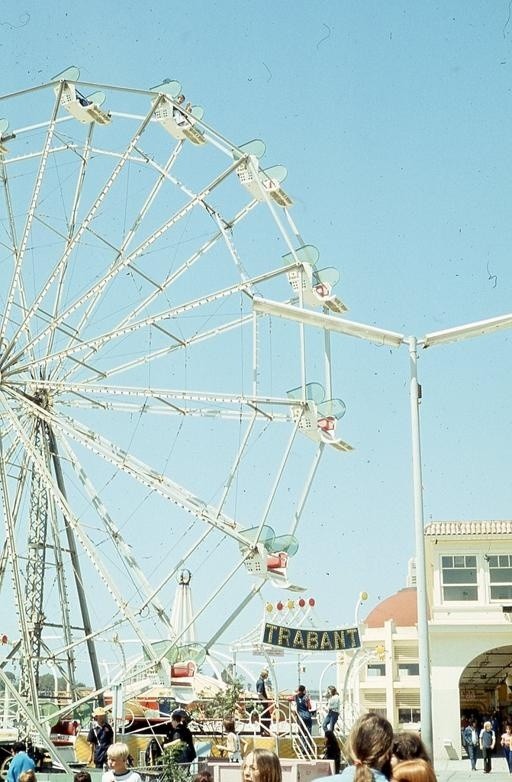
[250,291,512,772]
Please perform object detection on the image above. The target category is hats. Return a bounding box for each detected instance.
[172,710,189,720]
[91,707,108,717]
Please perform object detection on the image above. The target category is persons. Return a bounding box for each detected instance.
[1,707,196,782]
[459,712,512,774]
[308,712,436,781]
[256,671,340,735]
[240,749,282,782]
